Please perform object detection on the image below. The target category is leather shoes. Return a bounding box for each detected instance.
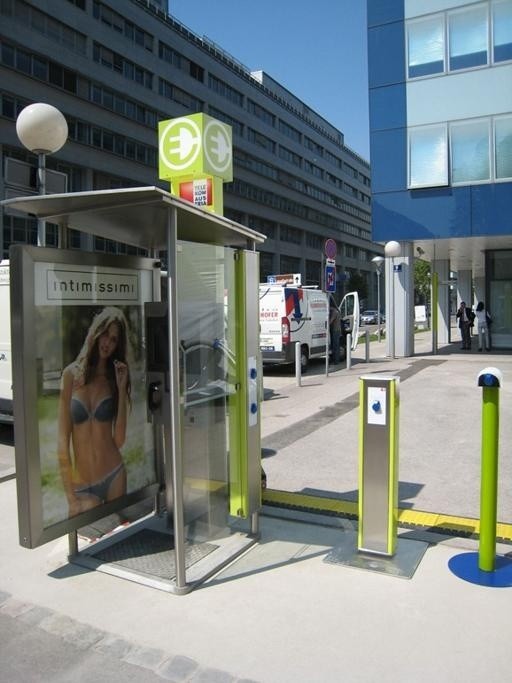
[461,346,490,351]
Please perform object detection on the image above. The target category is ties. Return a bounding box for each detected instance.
[462,310,464,321]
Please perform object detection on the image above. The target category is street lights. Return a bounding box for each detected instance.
[370,255,386,342]
[424,270,431,329]
[11,101,73,563]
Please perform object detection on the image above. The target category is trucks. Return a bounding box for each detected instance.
[1,258,236,440]
[219,272,362,374]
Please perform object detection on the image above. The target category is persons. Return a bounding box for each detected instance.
[475,300,491,352]
[455,301,476,350]
[329,304,341,364]
[58,304,134,517]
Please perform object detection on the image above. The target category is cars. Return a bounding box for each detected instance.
[344,308,386,325]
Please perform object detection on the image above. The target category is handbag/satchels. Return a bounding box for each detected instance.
[485,310,492,324]
